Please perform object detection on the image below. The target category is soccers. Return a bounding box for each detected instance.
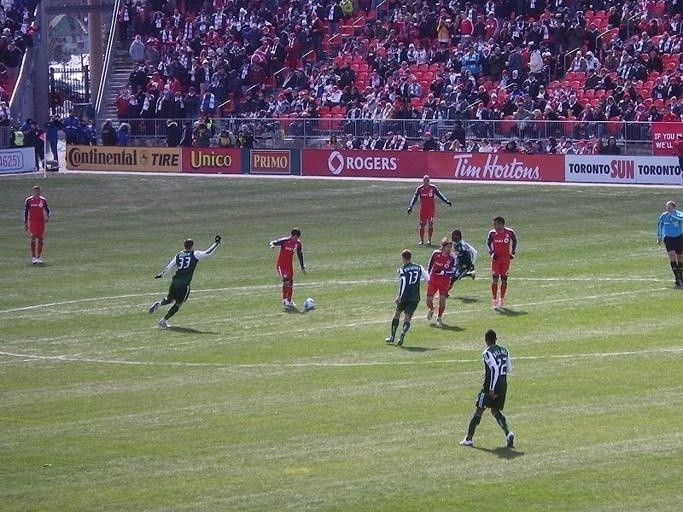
[304,298,315,310]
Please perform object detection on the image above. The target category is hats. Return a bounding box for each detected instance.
[439,238,455,250]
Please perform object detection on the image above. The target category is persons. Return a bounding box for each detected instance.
[656,201,683,288]
[407,174,452,246]
[459,329,515,448]
[24,185,51,264]
[106,0,683,155]
[486,216,517,311]
[426,237,456,328]
[0,0,105,171]
[433,229,478,299]
[268,228,309,308]
[384,249,430,346]
[148,234,222,328]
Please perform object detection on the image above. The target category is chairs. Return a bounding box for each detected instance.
[350,63,358,73]
[585,89,596,99]
[322,35,331,49]
[501,117,514,133]
[340,107,347,116]
[578,72,586,79]
[330,106,341,117]
[346,17,353,26]
[425,72,435,82]
[355,19,366,31]
[342,26,354,42]
[415,72,424,81]
[343,56,352,64]
[368,10,376,20]
[355,81,365,91]
[656,98,665,108]
[359,9,367,20]
[592,19,602,26]
[580,98,590,107]
[562,80,570,89]
[422,82,431,96]
[318,106,330,116]
[354,54,363,62]
[565,71,576,80]
[668,62,677,71]
[332,56,341,67]
[595,89,605,99]
[661,53,670,63]
[650,71,659,80]
[264,78,276,91]
[429,63,439,72]
[572,80,580,90]
[671,54,681,64]
[551,80,561,90]
[360,64,369,71]
[418,63,429,73]
[609,73,618,79]
[338,19,343,25]
[331,114,343,130]
[412,97,422,108]
[592,99,600,108]
[637,82,644,98]
[645,80,655,98]
[318,115,331,131]
[577,89,585,98]
[359,72,369,81]
[652,35,661,42]
[644,99,653,111]
[323,17,330,26]
[409,64,417,72]
[595,10,607,19]
[584,10,594,18]
[223,102,235,112]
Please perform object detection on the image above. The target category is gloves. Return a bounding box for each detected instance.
[490,253,496,262]
[445,200,452,207]
[407,208,412,215]
[510,254,515,259]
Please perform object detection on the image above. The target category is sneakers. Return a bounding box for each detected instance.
[459,438,473,446]
[398,337,403,345]
[418,240,424,246]
[674,280,681,288]
[284,300,292,308]
[427,308,435,320]
[385,335,394,342]
[492,299,499,309]
[427,240,431,245]
[505,432,514,447]
[498,299,504,306]
[158,320,169,327]
[435,319,447,329]
[36,258,43,263]
[31,257,37,263]
[149,301,160,313]
[470,270,475,281]
